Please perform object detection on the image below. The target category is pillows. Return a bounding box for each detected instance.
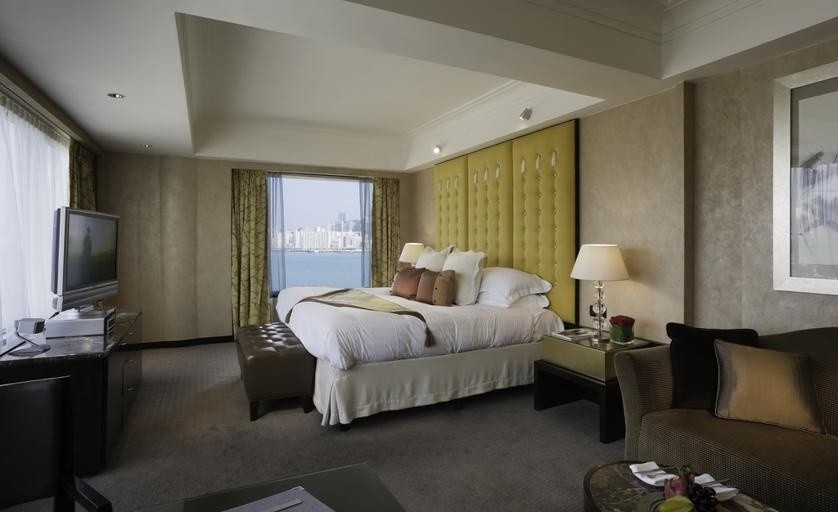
[389,267,425,301]
[475,267,552,308]
[666,322,759,409]
[416,270,455,306]
[415,245,455,272]
[442,248,487,305]
[713,339,825,435]
[512,295,549,309]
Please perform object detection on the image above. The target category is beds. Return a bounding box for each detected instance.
[276,245,565,427]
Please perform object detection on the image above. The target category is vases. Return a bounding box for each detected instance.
[610,326,635,345]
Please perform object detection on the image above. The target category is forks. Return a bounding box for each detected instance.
[703,473,733,488]
[649,470,680,478]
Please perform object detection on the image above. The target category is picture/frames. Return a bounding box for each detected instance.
[551,328,599,342]
[773,60,838,295]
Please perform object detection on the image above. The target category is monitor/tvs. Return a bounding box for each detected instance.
[51,206,121,312]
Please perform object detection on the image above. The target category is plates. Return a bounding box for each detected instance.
[635,489,701,512]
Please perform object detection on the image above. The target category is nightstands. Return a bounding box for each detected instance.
[534,330,668,444]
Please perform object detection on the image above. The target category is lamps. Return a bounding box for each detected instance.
[570,244,629,342]
[519,108,532,120]
[399,243,424,267]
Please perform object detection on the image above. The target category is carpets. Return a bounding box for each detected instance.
[0,342,625,512]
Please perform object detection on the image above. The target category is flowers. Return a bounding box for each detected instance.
[607,315,635,342]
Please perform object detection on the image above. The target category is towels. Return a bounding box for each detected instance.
[692,473,740,501]
[630,462,679,487]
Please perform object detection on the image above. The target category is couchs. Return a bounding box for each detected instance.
[614,322,838,512]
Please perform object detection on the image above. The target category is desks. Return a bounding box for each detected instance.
[135,461,411,512]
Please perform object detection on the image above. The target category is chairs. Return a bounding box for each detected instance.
[0,376,114,512]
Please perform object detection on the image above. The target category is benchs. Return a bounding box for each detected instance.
[236,322,317,422]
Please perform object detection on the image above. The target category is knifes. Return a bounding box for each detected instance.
[634,462,678,475]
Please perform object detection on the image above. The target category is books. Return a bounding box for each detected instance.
[552,328,598,341]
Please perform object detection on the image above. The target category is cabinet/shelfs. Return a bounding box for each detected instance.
[0,311,143,470]
